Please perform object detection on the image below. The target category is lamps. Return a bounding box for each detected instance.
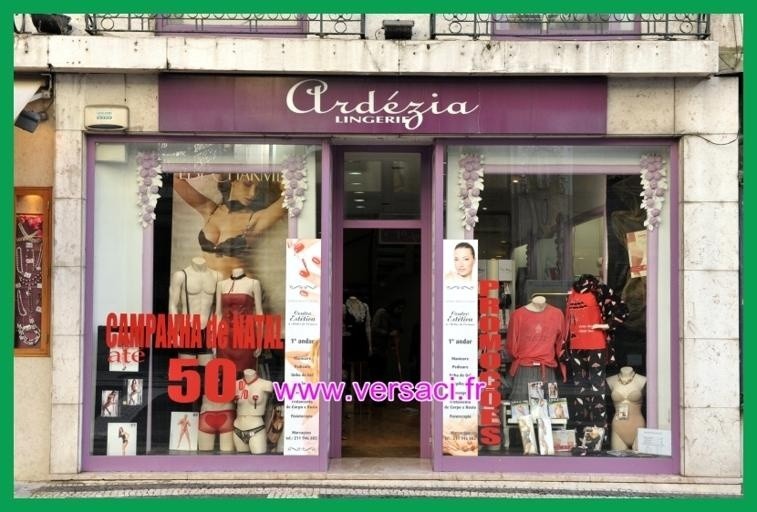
[12,110,49,135]
[377,16,416,41]
[29,13,74,36]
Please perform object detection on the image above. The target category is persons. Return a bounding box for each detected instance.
[102,173,287,454]
[454,242,474,278]
[366,296,409,382]
[343,296,372,402]
[507,274,646,456]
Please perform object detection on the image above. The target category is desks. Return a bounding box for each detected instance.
[499,396,569,450]
